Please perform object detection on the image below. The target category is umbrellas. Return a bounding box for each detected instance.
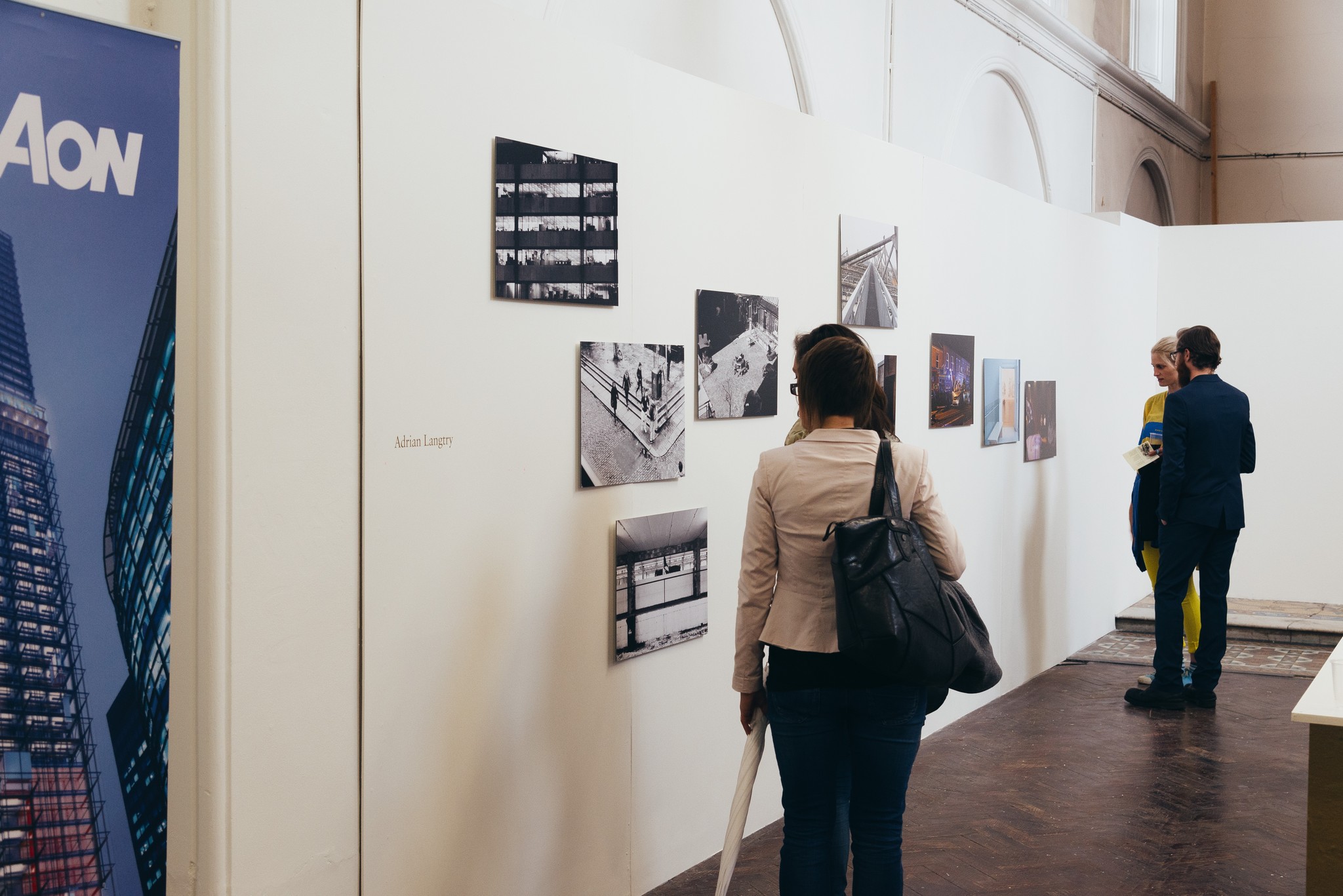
[715,663,769,896]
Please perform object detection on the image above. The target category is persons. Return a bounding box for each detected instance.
[636,362,643,397]
[648,394,657,443]
[1129,336,1201,686]
[611,381,619,423]
[641,389,650,433]
[784,324,900,447]
[556,226,559,231]
[622,370,631,411]
[546,226,548,230]
[732,336,965,896]
[525,251,527,265]
[1124,326,1256,711]
[562,226,565,231]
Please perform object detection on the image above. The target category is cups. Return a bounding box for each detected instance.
[1149,432,1162,451]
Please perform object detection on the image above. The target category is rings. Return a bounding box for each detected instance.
[1156,449,1159,454]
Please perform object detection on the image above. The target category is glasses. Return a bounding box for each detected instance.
[790,382,798,397]
[1169,349,1195,361]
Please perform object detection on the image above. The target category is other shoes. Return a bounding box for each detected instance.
[1182,668,1192,686]
[1184,683,1217,709]
[1138,672,1157,684]
[1124,688,1187,711]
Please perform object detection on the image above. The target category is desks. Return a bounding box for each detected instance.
[1291,635,1343,896]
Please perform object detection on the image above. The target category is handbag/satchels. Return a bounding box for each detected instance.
[820,440,1003,695]
